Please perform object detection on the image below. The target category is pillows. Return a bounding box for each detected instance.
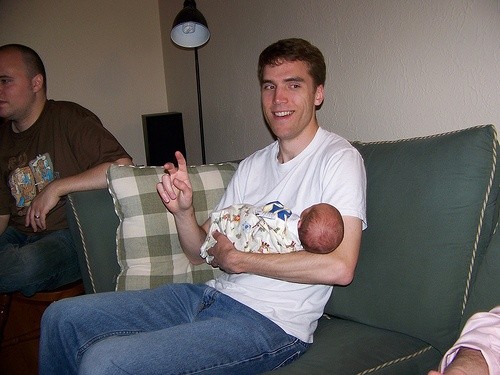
[103,159,239,293]
[323,123,500,356]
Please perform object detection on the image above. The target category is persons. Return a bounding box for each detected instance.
[199,200,344,263]
[428,303,500,375]
[39,38,367,375]
[1,44,135,294]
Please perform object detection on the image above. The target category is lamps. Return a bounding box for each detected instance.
[169,0,213,164]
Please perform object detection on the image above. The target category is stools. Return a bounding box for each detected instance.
[0,280,85,350]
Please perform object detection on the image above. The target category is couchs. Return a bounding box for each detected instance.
[67,159,500,375]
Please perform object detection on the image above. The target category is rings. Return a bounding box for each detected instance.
[34,215,40,219]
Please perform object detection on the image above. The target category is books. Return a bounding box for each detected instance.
[141,112,187,166]
